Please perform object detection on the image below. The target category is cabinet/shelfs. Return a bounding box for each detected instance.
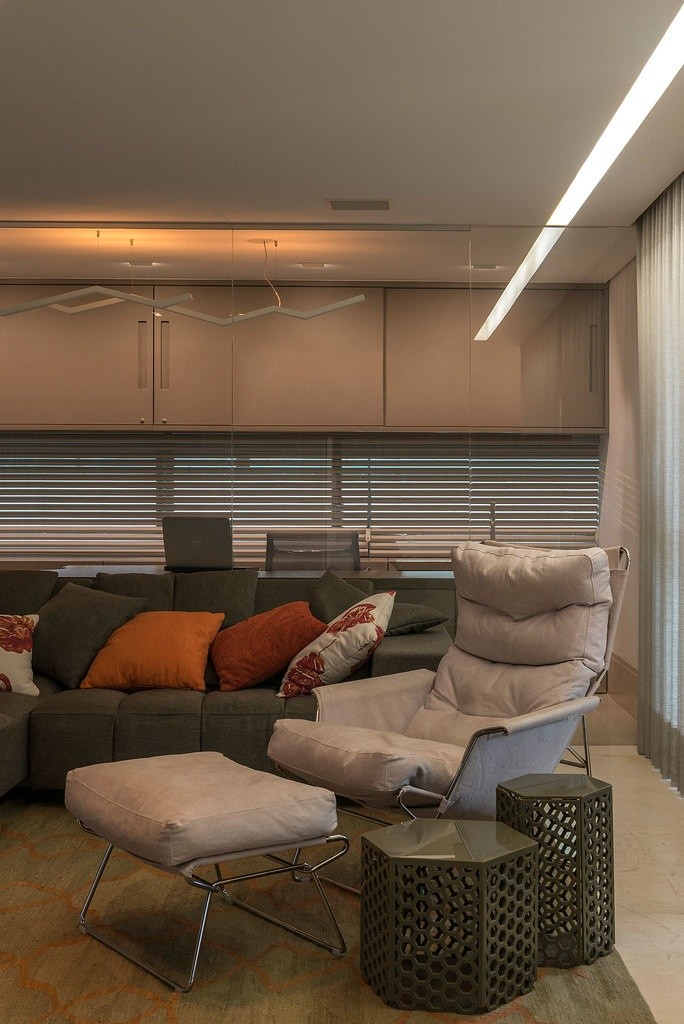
[0,278,610,434]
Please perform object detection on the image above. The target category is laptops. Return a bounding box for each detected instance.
[162,516,262,572]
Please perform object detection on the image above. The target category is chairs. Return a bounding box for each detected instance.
[265,530,362,570]
[265,539,632,896]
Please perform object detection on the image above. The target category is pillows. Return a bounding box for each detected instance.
[385,603,450,637]
[80,611,224,692]
[93,573,175,612]
[275,591,399,698]
[0,570,59,615]
[0,614,40,697]
[211,601,329,693]
[300,571,370,623]
[174,568,260,632]
[31,581,148,690]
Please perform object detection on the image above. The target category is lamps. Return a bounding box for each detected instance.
[0,230,364,326]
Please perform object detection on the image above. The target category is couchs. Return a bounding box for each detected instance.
[0,570,453,800]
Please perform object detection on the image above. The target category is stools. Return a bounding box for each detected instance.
[65,750,350,994]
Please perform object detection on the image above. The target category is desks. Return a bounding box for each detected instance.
[59,565,457,640]
